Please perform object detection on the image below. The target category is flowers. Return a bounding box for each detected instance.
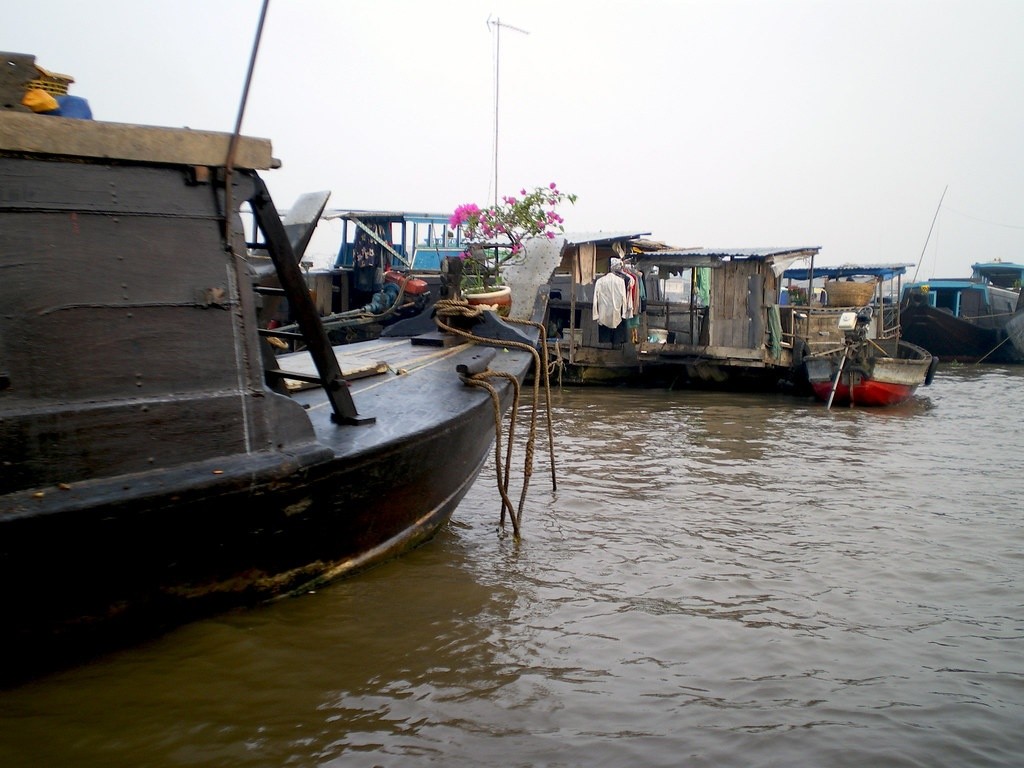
[448,181,579,293]
[789,285,803,295]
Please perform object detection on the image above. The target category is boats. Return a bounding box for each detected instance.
[0,0,556,689]
[240,185,1024,409]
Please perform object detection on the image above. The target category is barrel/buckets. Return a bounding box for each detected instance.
[647,328,669,345]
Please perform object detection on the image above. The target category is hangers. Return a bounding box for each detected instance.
[608,256,634,276]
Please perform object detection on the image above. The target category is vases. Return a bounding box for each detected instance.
[794,296,804,305]
[460,285,513,319]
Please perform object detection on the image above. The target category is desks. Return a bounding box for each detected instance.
[539,300,599,342]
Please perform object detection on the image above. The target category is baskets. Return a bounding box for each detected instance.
[23,72,74,96]
[825,281,875,307]
